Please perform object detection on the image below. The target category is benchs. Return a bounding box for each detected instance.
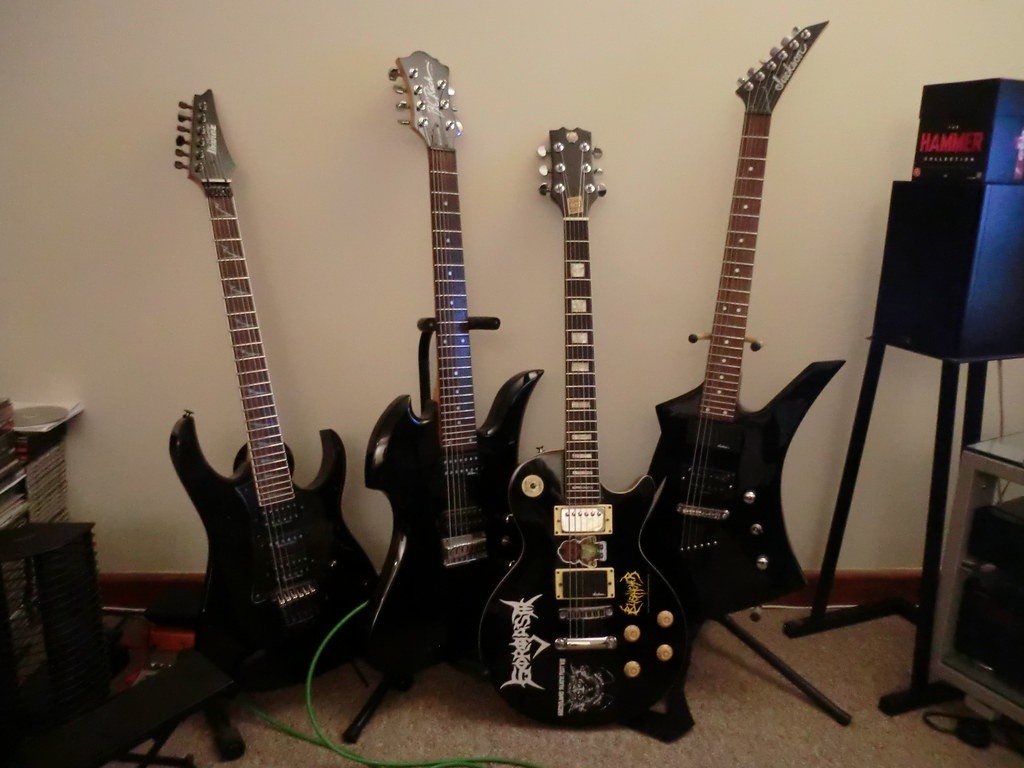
[0,653,233,768]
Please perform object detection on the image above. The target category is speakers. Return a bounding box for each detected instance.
[873,181,1024,362]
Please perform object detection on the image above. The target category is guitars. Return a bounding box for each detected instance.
[477,125,688,729]
[642,18,847,621]
[364,50,546,693]
[168,89,381,689]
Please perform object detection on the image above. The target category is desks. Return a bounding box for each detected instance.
[930,430,1024,725]
[782,337,1024,718]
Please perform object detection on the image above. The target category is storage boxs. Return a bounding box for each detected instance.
[912,79,1024,185]
[872,181,1024,358]
